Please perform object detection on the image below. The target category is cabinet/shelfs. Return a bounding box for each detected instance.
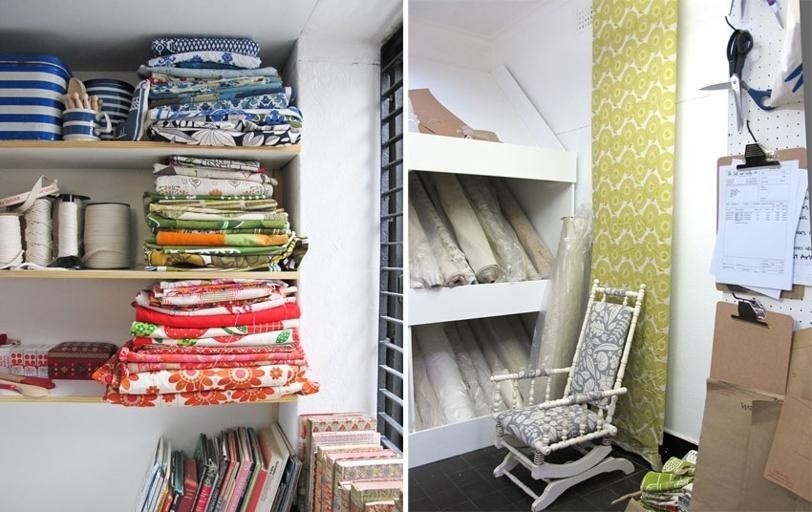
[403,131,578,471]
[0,139,301,404]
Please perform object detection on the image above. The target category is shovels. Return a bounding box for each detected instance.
[0,379,49,397]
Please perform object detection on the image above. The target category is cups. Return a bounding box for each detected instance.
[62,109,111,140]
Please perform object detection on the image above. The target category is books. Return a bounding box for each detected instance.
[134,412,403,512]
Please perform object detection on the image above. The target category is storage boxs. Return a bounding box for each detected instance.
[0,55,69,141]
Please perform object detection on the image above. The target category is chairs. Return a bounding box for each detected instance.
[491,278,645,512]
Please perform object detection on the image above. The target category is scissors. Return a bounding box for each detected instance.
[784,63,803,93]
[699,29,778,111]
[727,30,754,132]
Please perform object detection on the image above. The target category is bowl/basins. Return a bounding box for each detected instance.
[84,77,134,140]
